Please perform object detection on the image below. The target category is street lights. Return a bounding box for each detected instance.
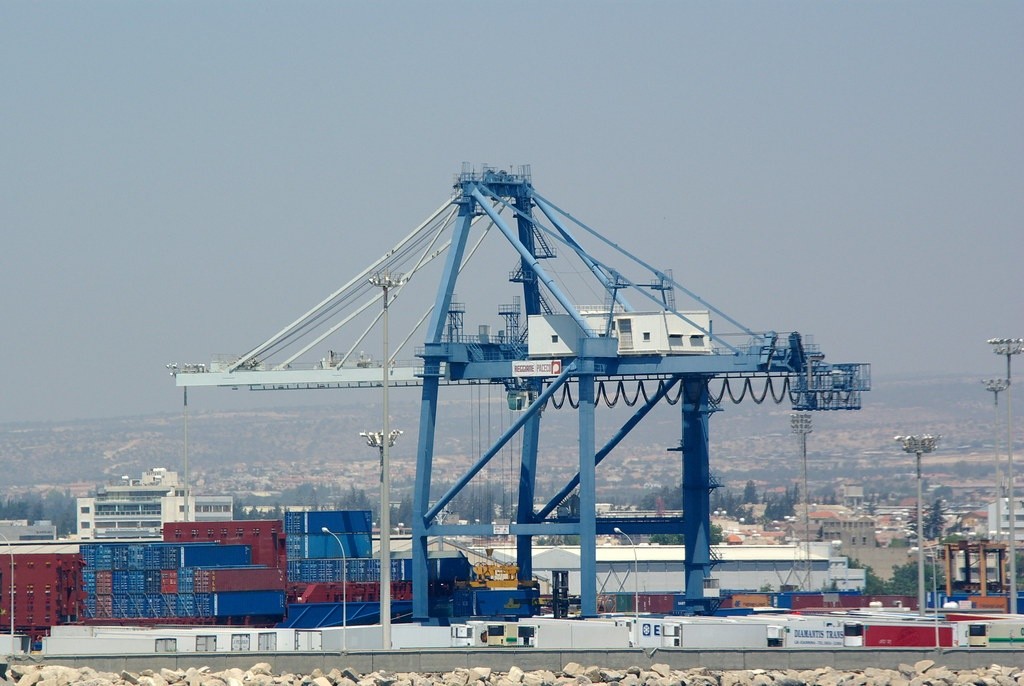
[321,526,347,627]
[979,377,1006,582]
[357,430,404,625]
[893,433,944,615]
[788,412,813,591]
[613,527,638,620]
[368,268,409,649]
[987,337,1024,614]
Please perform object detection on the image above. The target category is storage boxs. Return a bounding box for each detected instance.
[73,510,542,617]
[603,588,1024,613]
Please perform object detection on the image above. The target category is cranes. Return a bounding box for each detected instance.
[167,161,872,627]
